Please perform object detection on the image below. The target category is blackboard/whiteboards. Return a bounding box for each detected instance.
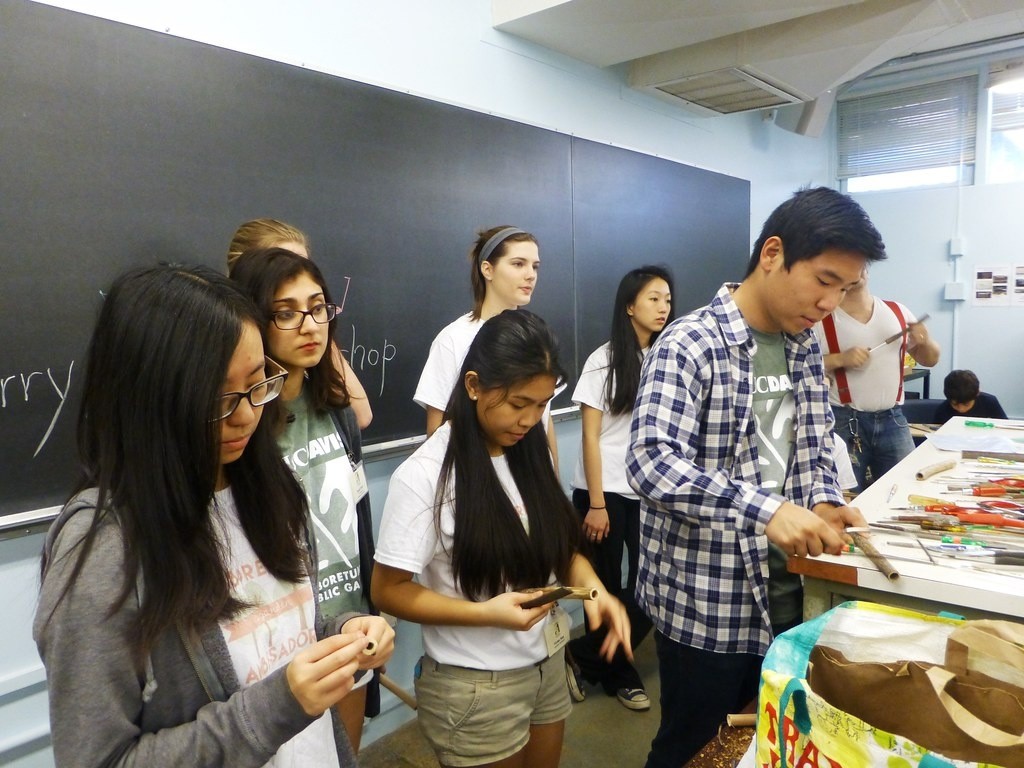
[0,2,754,539]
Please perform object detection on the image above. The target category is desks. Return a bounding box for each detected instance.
[786,415,1024,623]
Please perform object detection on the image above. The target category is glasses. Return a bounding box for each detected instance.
[197,354,289,424]
[268,302,337,330]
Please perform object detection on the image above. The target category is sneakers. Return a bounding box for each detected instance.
[564,645,585,701]
[617,687,650,709]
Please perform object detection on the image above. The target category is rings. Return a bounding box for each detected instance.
[920,334,923,338]
[593,534,596,536]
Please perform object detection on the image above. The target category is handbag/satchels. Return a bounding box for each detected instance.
[754,601,1024,768]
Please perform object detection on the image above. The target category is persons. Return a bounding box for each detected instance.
[370,311,632,768]
[33,187,1008,767]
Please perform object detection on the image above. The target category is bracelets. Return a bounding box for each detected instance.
[590,506,606,509]
